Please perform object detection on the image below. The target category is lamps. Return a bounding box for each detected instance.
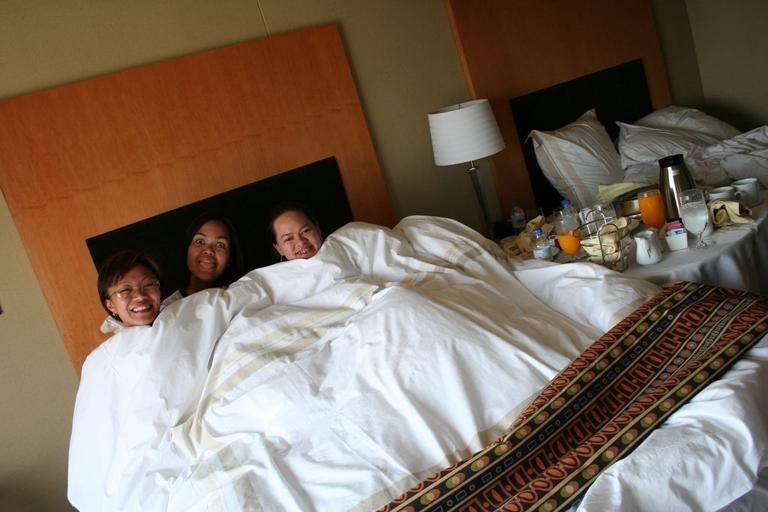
[427,97,508,244]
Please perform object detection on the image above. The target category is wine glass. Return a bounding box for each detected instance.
[638,190,713,249]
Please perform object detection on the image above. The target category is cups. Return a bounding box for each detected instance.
[710,178,763,209]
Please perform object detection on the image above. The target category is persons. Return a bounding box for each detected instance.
[254,204,328,262]
[96,250,165,336]
[160,212,245,316]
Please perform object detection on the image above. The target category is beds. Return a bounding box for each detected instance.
[65,258,766,511]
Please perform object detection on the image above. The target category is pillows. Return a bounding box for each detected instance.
[523,106,745,212]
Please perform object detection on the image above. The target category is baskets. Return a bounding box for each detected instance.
[571,210,633,272]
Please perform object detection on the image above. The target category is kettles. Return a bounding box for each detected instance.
[658,153,696,224]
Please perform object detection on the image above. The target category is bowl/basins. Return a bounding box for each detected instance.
[613,184,659,217]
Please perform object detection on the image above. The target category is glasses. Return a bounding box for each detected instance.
[108,277,161,299]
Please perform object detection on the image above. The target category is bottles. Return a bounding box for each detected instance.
[510,202,527,234]
[633,228,663,266]
[533,199,612,262]
